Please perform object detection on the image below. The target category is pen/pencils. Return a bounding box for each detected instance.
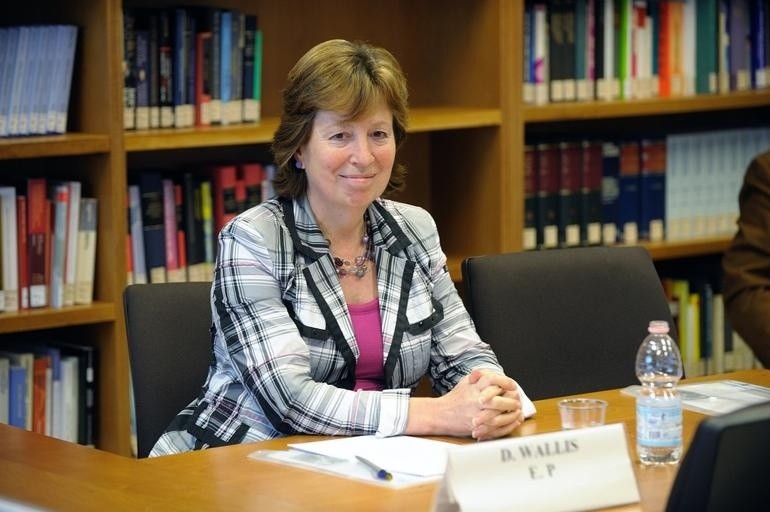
[355,455,392,481]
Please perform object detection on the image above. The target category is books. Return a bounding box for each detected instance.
[519,2,770,252]
[662,279,764,378]
[1,6,277,447]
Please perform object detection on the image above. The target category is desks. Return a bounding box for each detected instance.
[1,369,770,511]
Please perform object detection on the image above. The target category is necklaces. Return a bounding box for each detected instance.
[317,212,377,277]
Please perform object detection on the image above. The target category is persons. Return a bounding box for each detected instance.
[148,37,536,458]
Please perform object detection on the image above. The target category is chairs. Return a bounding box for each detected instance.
[462,246,685,402]
[665,403,770,511]
[124,284,212,456]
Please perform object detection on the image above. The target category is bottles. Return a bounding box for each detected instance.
[635,320,683,466]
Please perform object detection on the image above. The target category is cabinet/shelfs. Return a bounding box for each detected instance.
[0,0,770,458]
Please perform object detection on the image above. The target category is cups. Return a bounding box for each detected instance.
[558,399,608,429]
